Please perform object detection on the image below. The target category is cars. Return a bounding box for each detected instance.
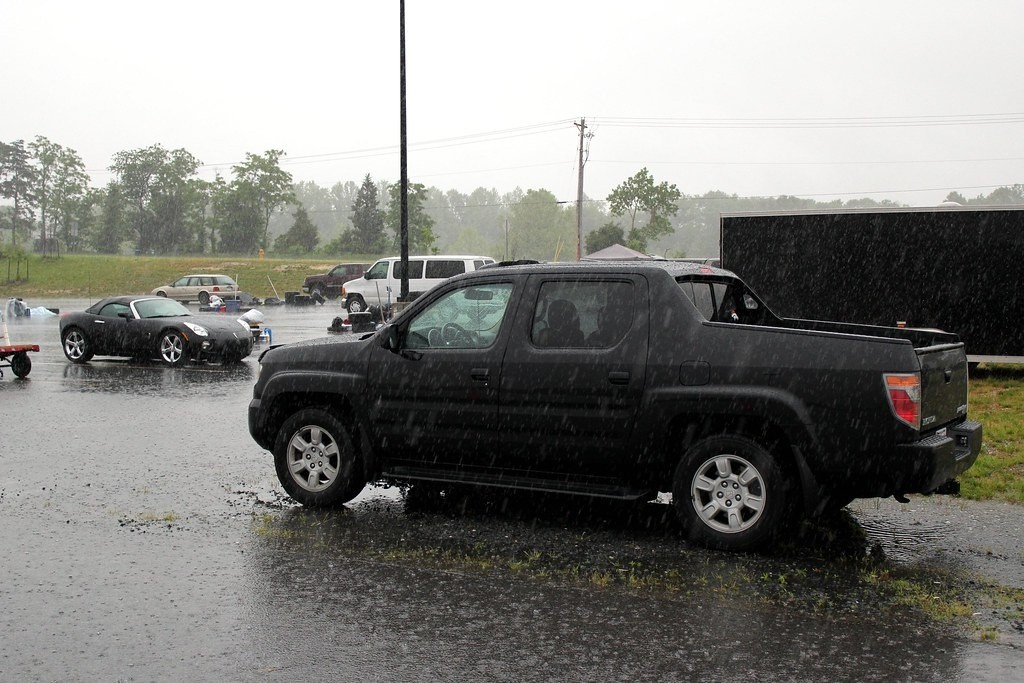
[149,274,242,304]
[58,295,254,367]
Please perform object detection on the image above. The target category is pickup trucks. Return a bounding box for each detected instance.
[248,256,984,553]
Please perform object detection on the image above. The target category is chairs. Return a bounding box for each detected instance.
[537,300,613,348]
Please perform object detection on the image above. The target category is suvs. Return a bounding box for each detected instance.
[304,263,377,301]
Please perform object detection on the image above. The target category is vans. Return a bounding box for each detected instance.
[339,255,505,323]
